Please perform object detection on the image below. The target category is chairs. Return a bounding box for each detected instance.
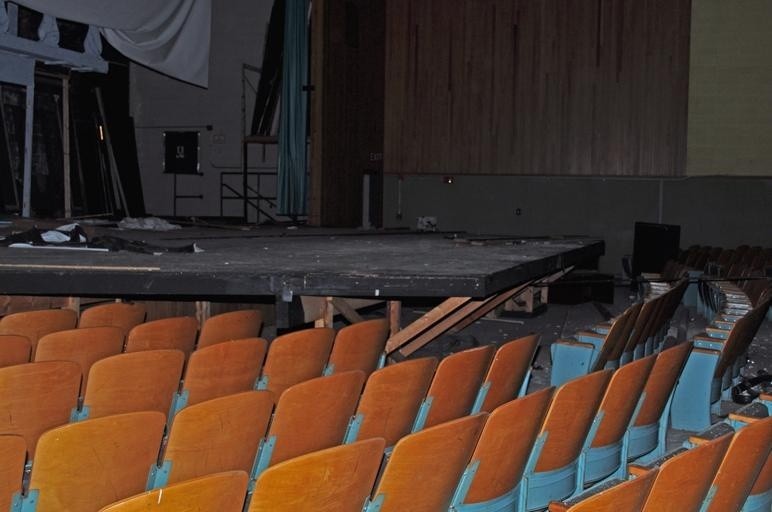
[0,242,771,512]
